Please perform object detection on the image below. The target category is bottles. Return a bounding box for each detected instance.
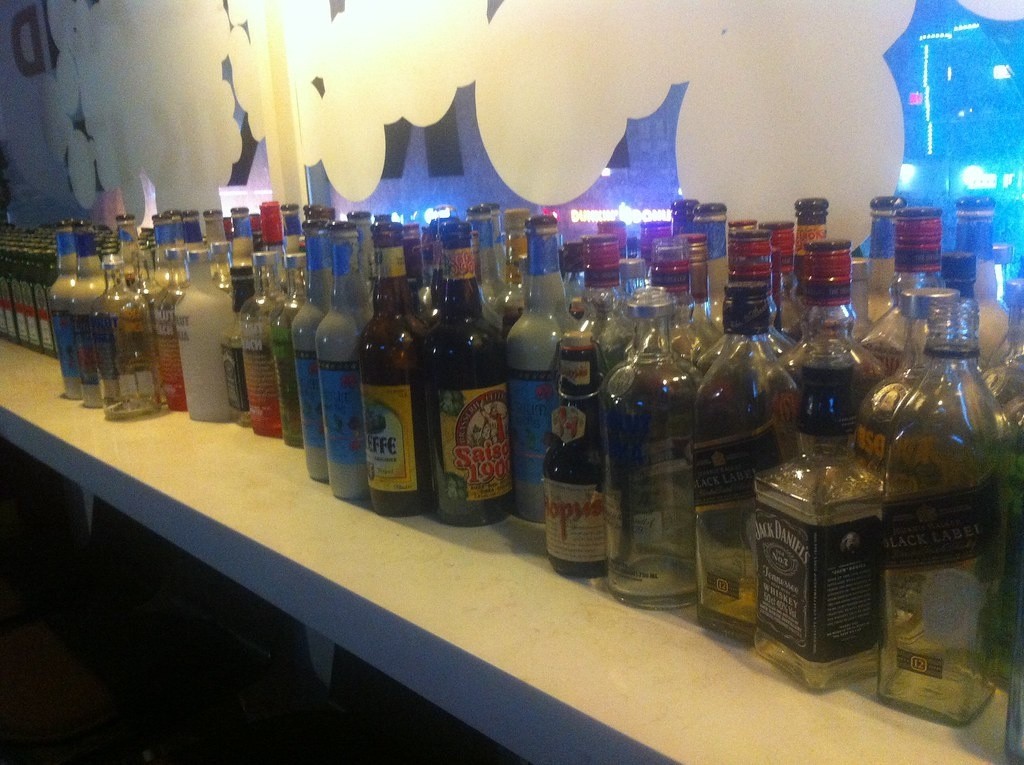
[0,200,1024,765]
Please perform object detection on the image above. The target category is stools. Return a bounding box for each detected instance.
[0,483,493,765]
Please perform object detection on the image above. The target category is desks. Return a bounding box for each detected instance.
[0,335,1022,765]
